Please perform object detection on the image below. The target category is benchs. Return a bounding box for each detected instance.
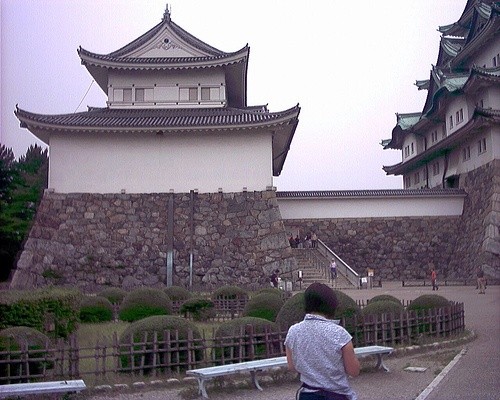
[0,379,86,398]
[186,356,288,398]
[354,345,394,372]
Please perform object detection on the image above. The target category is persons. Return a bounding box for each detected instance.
[304,232,311,248]
[270,269,280,289]
[477,265,487,294]
[330,258,337,278]
[311,231,317,248]
[431,269,439,291]
[284,283,360,400]
[290,235,303,248]
